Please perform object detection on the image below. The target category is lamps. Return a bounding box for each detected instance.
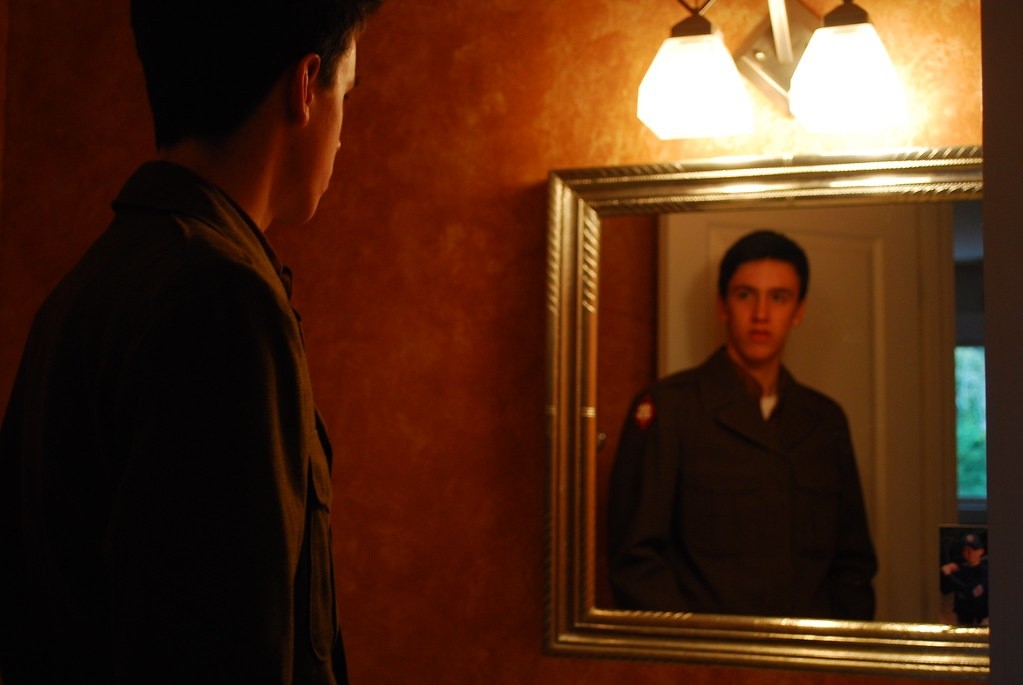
[636,0,890,140]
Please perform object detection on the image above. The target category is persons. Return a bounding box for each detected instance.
[940,534,988,627]
[607,230,877,622]
[0,1,383,684]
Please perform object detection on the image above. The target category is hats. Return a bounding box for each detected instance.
[961,533,982,550]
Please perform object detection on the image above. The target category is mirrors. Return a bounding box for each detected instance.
[550,145,991,679]
